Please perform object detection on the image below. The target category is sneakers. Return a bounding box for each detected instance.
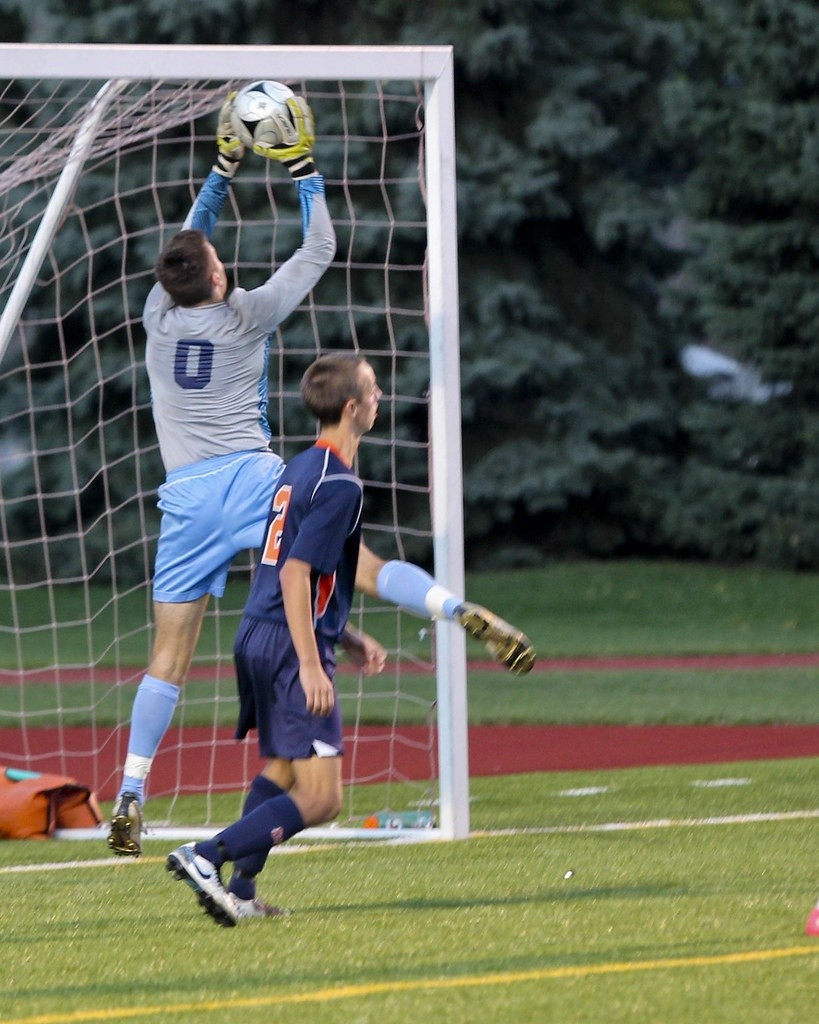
[225,891,291,918]
[166,842,240,927]
[108,792,141,857]
[453,600,537,677]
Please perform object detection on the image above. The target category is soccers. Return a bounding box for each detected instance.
[229,81,304,150]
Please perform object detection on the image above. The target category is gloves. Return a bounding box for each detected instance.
[211,90,245,179]
[252,96,320,180]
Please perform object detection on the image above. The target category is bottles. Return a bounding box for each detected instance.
[365,812,431,828]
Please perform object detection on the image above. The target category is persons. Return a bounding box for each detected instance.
[102,88,532,857]
[164,348,385,931]
